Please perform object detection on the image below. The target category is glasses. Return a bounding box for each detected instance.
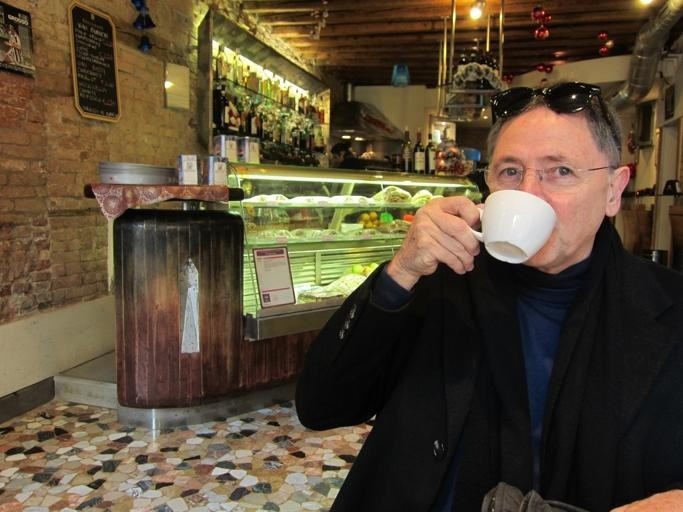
[476,156,612,192]
[490,81,613,127]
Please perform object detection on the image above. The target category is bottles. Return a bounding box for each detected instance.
[213,43,324,151]
[401,130,437,175]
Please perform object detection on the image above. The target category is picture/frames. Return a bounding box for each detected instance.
[422,107,457,151]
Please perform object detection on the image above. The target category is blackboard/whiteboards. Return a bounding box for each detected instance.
[67,1,122,123]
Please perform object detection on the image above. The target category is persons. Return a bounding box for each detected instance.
[0,23,24,66]
[291,81,682,512]
[329,140,358,169]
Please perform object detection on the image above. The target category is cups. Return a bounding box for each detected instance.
[461,190,555,263]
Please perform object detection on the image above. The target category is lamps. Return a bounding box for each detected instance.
[470,0,487,19]
[131,0,156,52]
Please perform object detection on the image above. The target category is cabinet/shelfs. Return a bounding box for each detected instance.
[199,161,491,341]
[197,7,332,167]
